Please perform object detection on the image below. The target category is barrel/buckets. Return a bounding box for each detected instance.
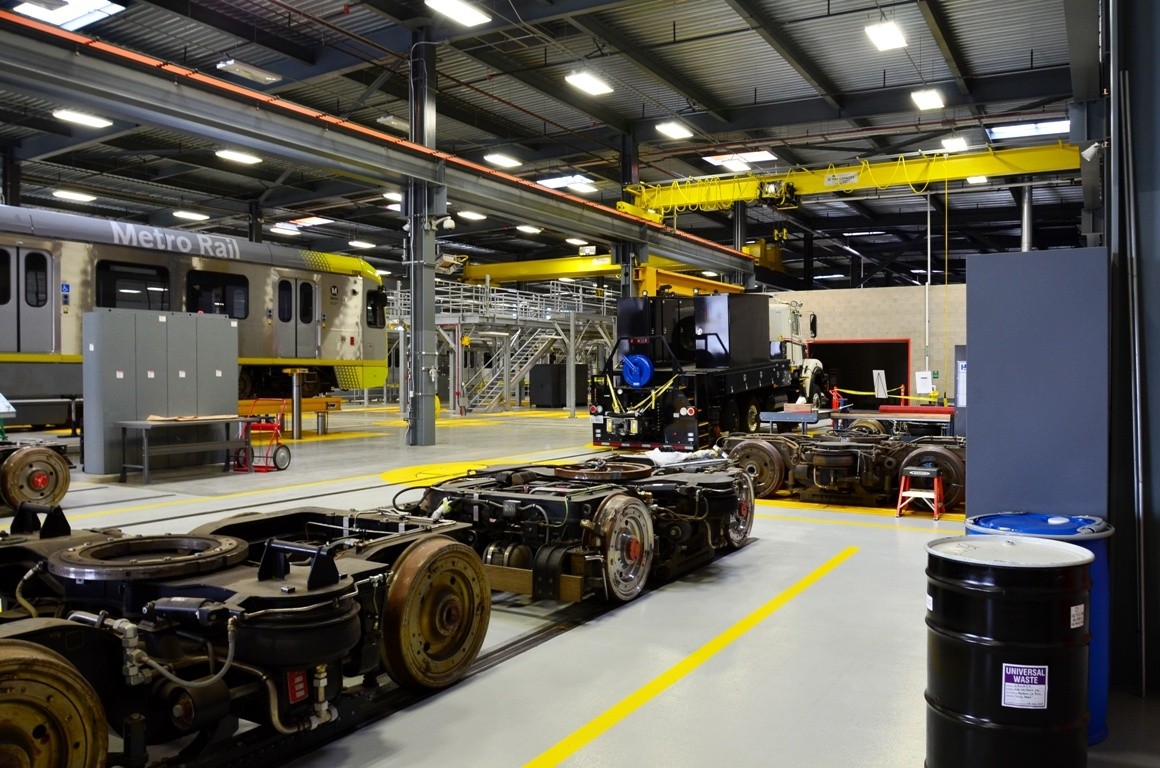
[966,512,1117,745]
[923,535,1095,768]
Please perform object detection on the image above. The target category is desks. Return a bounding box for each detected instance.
[115,416,260,485]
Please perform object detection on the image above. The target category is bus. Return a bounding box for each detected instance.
[0,205,391,434]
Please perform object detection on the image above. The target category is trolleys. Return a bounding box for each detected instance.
[233,397,293,472]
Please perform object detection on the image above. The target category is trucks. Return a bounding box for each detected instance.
[590,284,830,451]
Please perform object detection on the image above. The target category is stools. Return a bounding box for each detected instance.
[897,468,945,521]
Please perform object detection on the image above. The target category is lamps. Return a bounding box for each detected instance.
[13,0,1100,247]
